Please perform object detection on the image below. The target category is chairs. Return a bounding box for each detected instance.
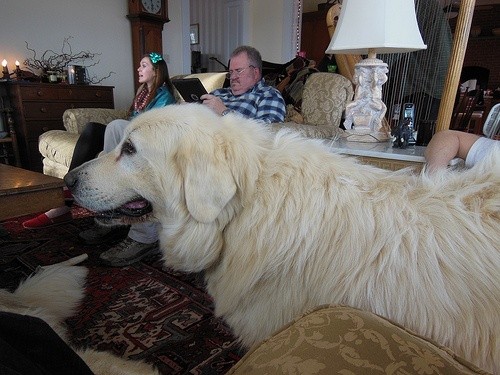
[450,85,481,131]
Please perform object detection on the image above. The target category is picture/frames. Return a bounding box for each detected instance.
[191,23,199,45]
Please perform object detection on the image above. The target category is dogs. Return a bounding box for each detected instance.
[63,102,500,375]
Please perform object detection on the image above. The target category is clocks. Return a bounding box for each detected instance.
[126,0,171,93]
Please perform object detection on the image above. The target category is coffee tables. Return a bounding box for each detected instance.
[0,163,65,253]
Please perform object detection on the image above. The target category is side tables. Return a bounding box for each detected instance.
[327,140,426,175]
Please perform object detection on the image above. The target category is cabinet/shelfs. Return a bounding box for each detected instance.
[2,82,115,173]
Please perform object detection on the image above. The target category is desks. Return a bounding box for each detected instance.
[456,111,485,134]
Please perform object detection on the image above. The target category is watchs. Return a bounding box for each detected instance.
[222,109,231,116]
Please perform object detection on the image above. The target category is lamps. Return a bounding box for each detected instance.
[2,58,23,84]
[325,0,428,145]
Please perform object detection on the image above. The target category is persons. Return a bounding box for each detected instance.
[100,45,285,266]
[426,130,500,181]
[24,51,175,230]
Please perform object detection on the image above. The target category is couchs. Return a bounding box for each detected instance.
[38,71,353,179]
[226,303,493,375]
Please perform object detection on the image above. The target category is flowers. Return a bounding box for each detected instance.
[149,52,163,63]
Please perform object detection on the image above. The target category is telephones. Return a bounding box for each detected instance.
[400,103,417,143]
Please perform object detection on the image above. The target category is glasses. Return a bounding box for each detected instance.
[225,66,255,79]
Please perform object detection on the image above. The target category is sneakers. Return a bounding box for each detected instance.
[100,236,160,265]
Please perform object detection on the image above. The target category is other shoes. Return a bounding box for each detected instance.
[22,211,72,229]
